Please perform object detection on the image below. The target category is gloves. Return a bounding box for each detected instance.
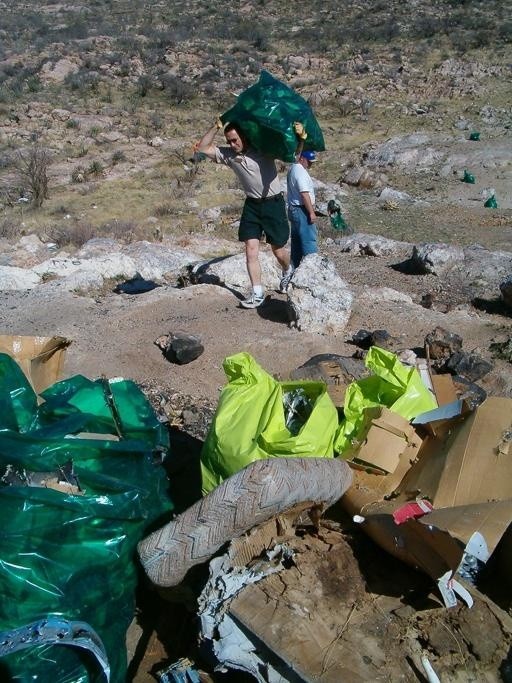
[293,121,307,141]
[215,112,224,128]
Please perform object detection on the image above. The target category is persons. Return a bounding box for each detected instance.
[196,118,308,308]
[288,150,319,267]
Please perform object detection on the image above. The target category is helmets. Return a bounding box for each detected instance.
[301,150,318,163]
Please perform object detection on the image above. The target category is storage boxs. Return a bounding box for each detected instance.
[0,334,74,395]
[337,342,512,588]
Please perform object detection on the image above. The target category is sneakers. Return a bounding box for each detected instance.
[279,264,295,294]
[240,294,267,308]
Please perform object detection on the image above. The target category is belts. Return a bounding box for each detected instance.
[247,193,281,203]
